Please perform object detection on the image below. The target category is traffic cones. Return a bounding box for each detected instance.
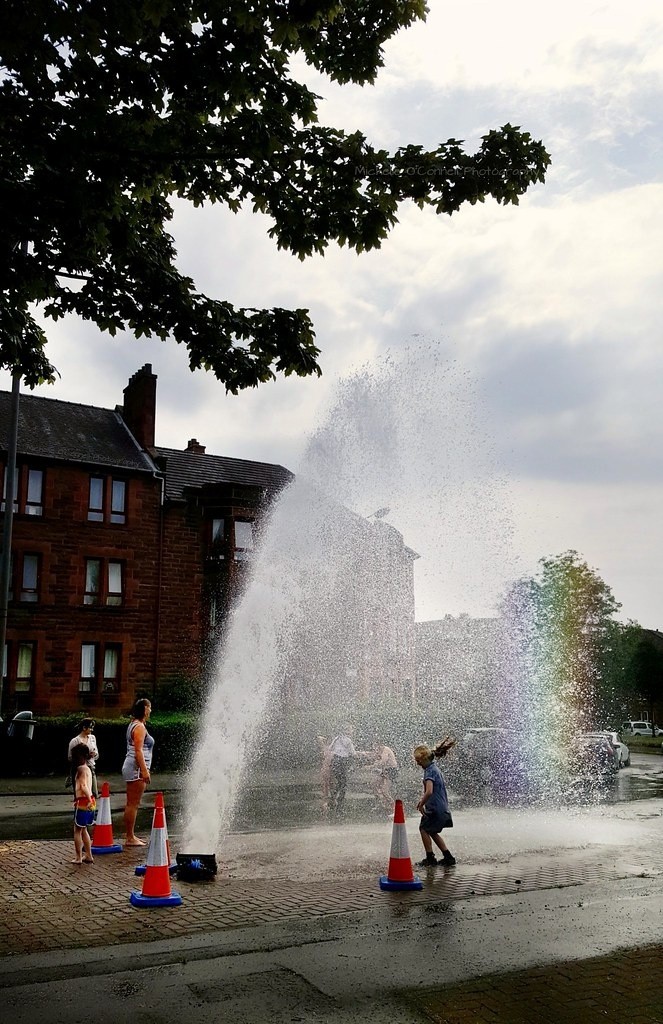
[130,808,183,908]
[378,799,425,892]
[81,782,124,855]
[133,792,179,877]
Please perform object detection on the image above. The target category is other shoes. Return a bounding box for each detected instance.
[437,857,456,867]
[415,857,438,867]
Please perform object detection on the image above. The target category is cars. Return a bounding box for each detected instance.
[621,721,663,737]
[448,726,632,788]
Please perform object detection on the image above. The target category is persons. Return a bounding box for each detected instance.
[314,723,400,819]
[413,736,459,867]
[67,716,99,864]
[121,698,155,847]
